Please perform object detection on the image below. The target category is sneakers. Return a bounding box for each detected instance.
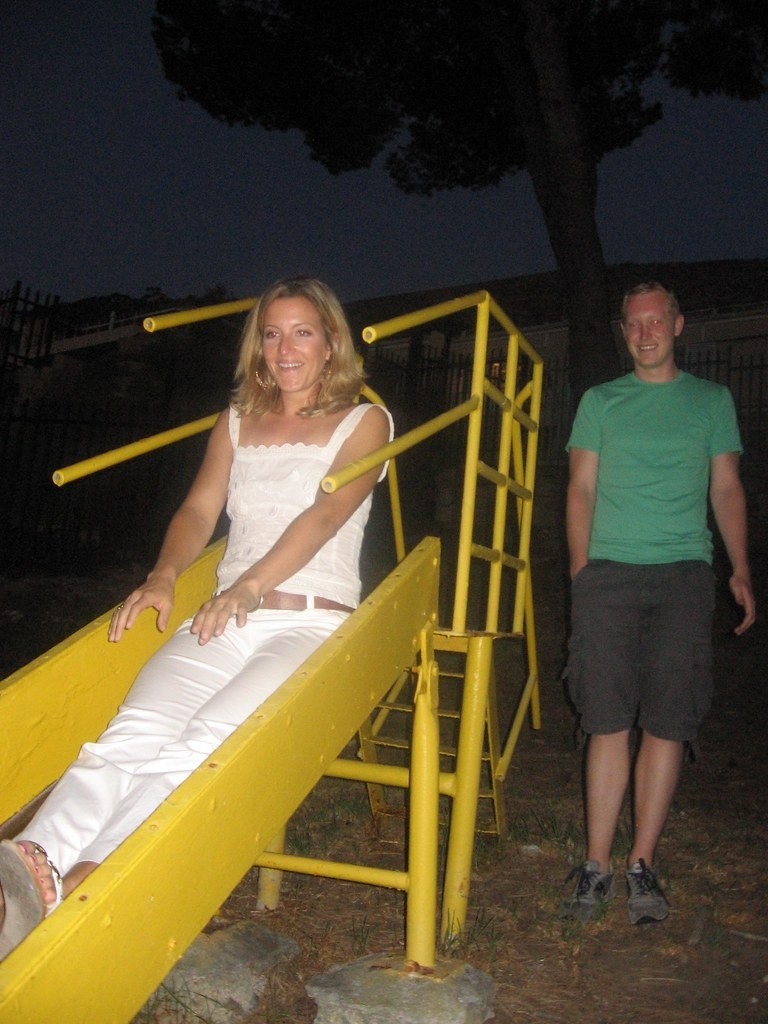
[626,857,671,927]
[564,859,616,922]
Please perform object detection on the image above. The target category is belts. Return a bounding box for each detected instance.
[214,589,356,613]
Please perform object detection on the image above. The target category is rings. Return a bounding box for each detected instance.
[118,604,124,609]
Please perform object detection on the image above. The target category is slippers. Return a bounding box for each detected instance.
[1,839,62,961]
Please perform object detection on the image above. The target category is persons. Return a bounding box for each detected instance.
[563,282,756,925]
[0,276,393,958]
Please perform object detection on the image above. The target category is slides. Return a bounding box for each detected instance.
[0,530,447,1024]
